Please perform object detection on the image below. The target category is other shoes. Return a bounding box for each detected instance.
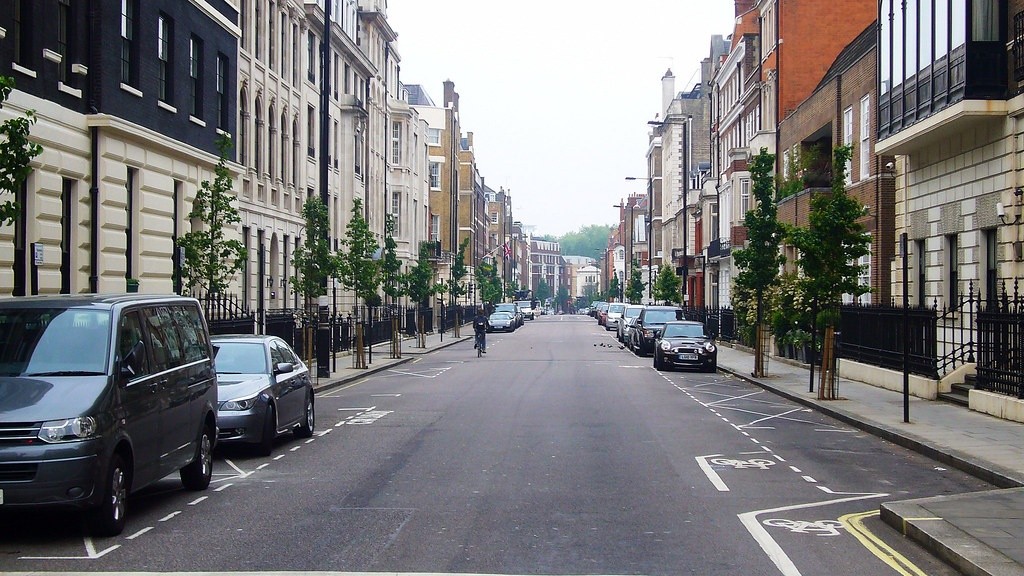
[474,343,478,349]
[482,351,486,353]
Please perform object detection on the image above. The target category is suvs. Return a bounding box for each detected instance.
[626,305,687,357]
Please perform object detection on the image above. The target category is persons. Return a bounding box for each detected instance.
[473,309,490,353]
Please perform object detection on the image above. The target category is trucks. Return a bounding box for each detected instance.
[512,299,542,320]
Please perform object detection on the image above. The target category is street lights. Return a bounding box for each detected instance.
[647,120,687,296]
[625,176,653,303]
[614,205,633,279]
[595,247,609,297]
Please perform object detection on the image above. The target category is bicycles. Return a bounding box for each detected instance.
[475,328,488,357]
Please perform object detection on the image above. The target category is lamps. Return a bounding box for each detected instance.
[996,203,1020,225]
[267,275,274,287]
[279,277,287,288]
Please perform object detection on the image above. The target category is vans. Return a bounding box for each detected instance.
[605,303,631,330]
[617,305,647,345]
[0,294,218,535]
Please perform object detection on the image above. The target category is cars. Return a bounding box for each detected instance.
[653,321,717,373]
[485,300,524,333]
[577,300,608,325]
[210,335,315,456]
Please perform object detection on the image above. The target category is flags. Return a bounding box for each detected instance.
[503,241,512,260]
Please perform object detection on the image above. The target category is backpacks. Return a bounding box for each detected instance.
[475,316,487,331]
